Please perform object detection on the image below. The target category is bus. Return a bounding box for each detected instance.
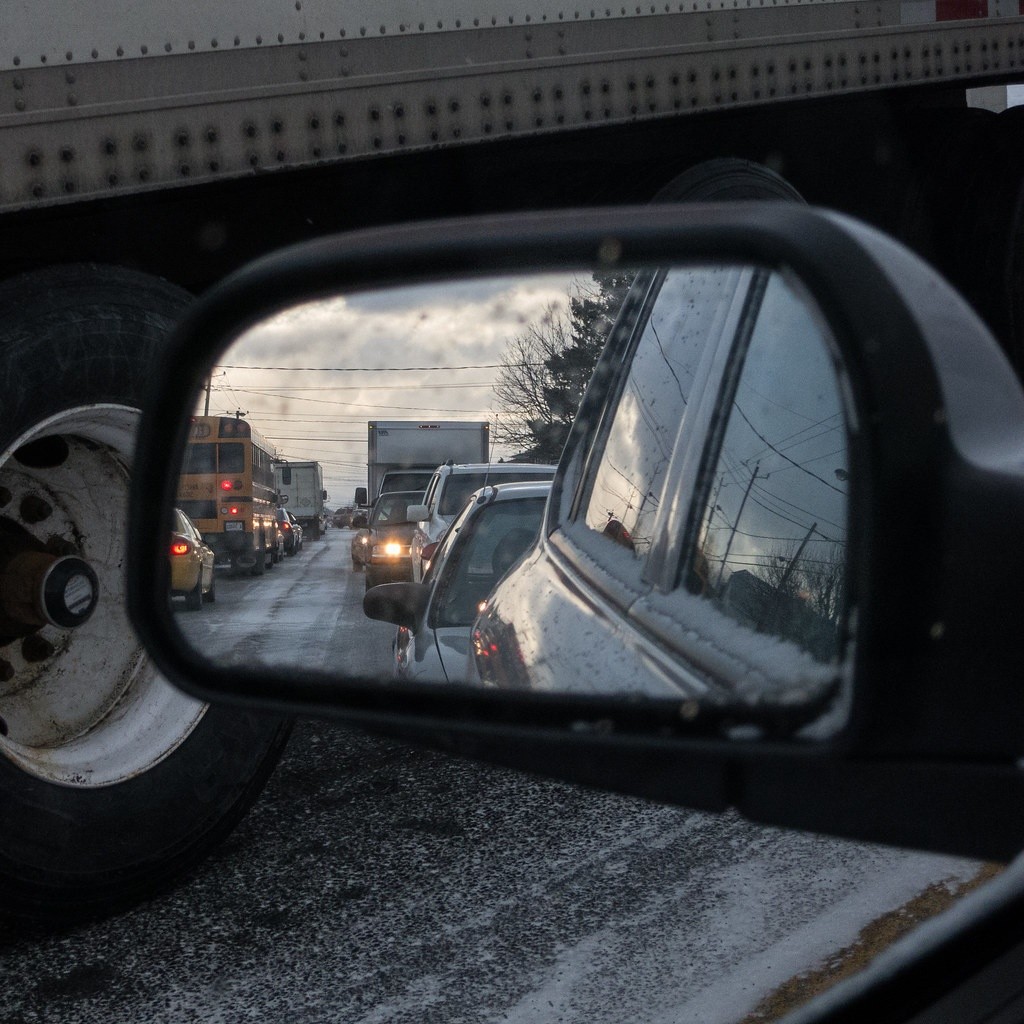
[179,415,291,576]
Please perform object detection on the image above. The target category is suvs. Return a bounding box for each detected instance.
[407,460,558,582]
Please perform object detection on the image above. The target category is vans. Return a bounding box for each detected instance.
[333,506,352,529]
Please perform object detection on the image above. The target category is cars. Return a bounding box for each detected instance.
[172,509,215,609]
[277,508,298,555]
[364,482,553,685]
[288,511,303,551]
[353,491,425,590]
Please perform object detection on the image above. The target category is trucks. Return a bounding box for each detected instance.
[368,421,489,522]
[275,462,327,540]
[2,1,1024,921]
